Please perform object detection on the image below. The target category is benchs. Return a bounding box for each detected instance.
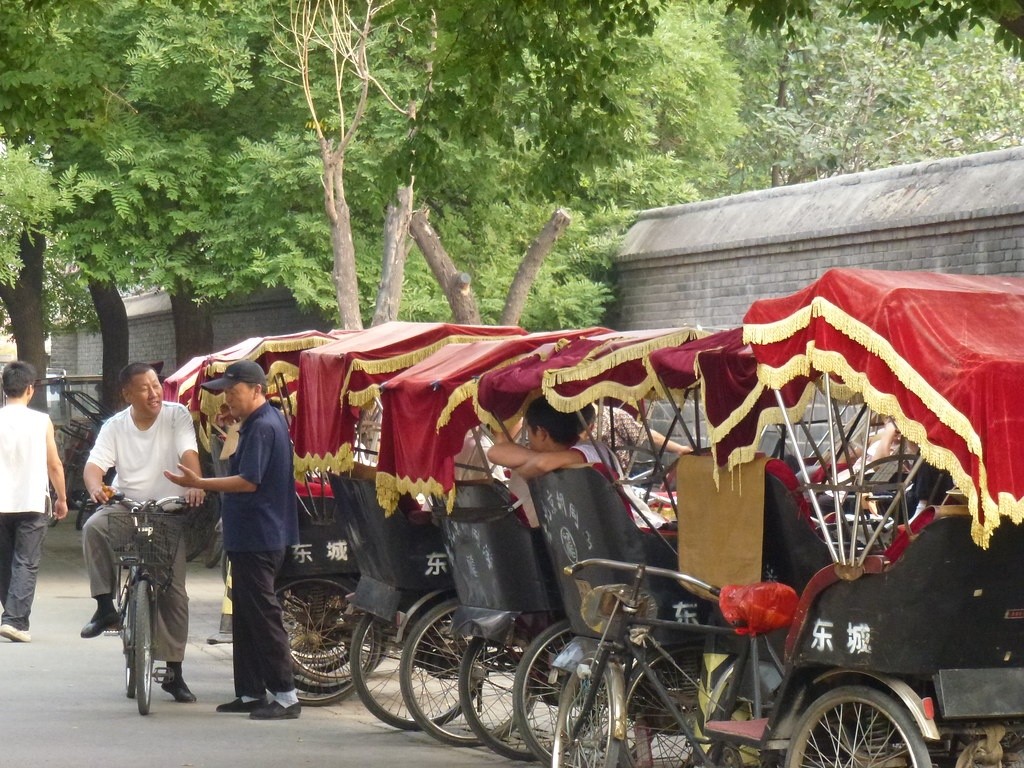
[327,468,452,588]
[534,465,704,646]
[206,432,360,575]
[785,505,1024,673]
[433,478,565,613]
[677,456,834,597]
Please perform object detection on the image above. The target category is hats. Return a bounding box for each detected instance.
[200,358,266,396]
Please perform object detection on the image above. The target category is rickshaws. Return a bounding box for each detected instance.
[161,269,1024,768]
[26,366,137,532]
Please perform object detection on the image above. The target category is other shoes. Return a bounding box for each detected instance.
[80,611,120,638]
[216,697,269,713]
[249,700,302,720]
[0,624,32,641]
[160,676,197,703]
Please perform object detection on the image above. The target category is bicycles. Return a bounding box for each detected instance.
[94,485,209,715]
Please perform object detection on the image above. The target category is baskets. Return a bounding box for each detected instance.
[106,510,185,566]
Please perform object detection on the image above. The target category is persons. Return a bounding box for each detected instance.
[165,363,303,721]
[81,362,205,702]
[0,361,69,642]
[452,395,690,528]
[785,412,950,546]
